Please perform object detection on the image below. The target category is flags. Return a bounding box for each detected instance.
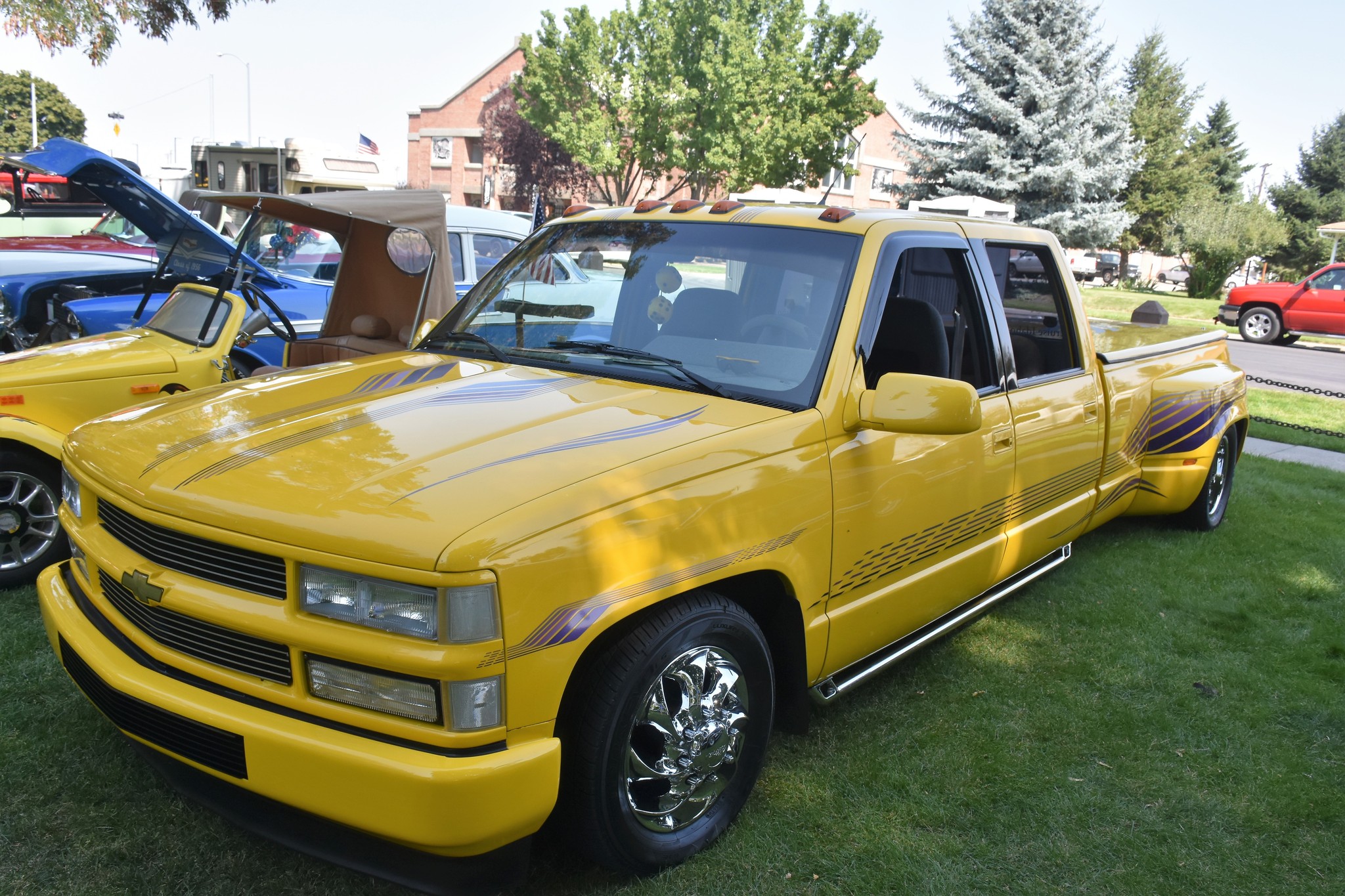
[359,134,380,156]
[526,193,557,288]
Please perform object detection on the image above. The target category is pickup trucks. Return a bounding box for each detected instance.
[28,191,1253,878]
[1009,247,1097,282]
[0,133,625,588]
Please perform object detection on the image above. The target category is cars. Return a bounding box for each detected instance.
[1217,262,1345,347]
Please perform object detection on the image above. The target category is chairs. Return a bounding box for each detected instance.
[659,288,745,343]
[865,297,950,379]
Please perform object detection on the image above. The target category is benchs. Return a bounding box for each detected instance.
[178,189,227,233]
[288,315,413,367]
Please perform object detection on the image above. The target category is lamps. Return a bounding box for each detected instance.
[484,175,491,205]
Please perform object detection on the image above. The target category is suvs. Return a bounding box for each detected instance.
[1083,253,1142,284]
[1155,264,1262,290]
